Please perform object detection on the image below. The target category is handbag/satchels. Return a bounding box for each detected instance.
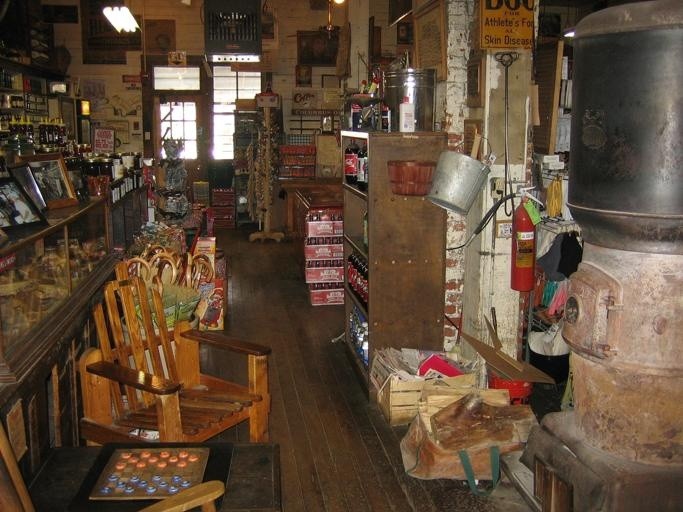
[400,394,540,481]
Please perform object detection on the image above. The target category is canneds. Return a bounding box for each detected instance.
[87,151,142,179]
[75,143,89,156]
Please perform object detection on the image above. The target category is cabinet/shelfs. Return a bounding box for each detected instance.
[0,197,120,491]
[290,187,343,263]
[341,130,448,383]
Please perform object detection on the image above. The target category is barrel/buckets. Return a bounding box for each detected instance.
[427,136,492,216]
[486,370,531,404]
[384,67,434,131]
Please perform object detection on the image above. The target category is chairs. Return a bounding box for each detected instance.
[1,425,225,512]
[79,261,272,446]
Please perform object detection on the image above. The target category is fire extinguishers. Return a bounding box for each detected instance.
[465,186,544,292]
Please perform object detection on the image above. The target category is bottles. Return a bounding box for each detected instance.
[67,143,144,200]
[349,304,368,372]
[347,249,368,314]
[345,139,368,191]
[359,78,379,94]
[306,211,343,289]
[362,209,368,253]
[399,96,415,132]
[12,95,24,108]
[9,115,67,147]
[0,70,12,88]
[382,107,391,133]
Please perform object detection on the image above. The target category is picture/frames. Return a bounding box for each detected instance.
[0,151,80,241]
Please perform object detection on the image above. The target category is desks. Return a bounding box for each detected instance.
[68,440,234,512]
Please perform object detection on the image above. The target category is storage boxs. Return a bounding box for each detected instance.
[304,210,344,306]
[373,351,480,426]
[212,188,235,230]
